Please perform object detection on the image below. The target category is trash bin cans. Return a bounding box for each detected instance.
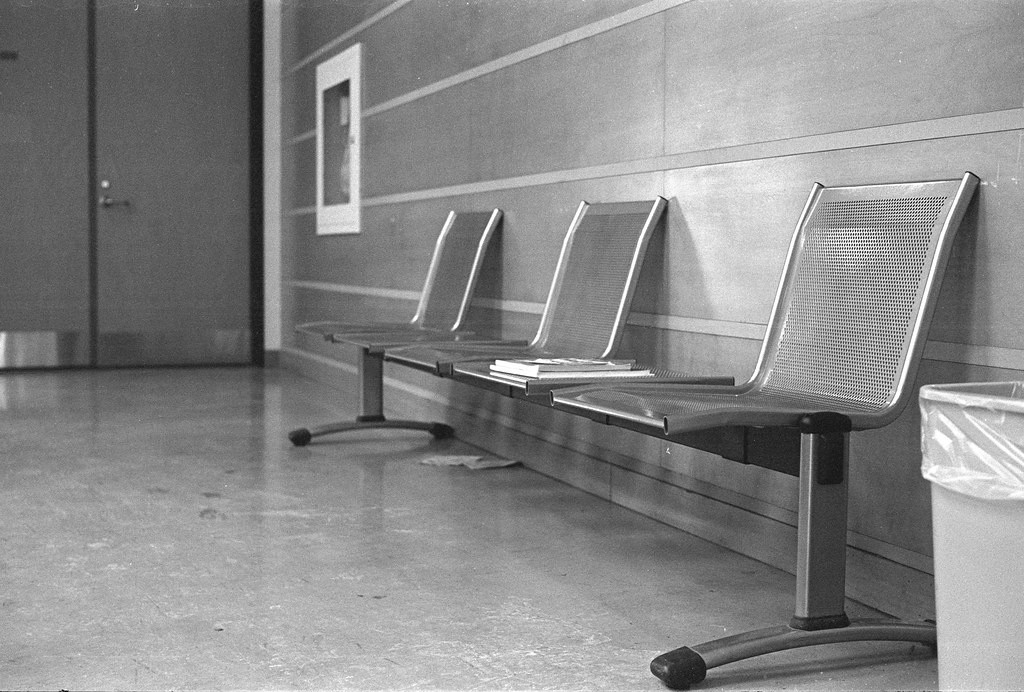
[918,376,1024,692]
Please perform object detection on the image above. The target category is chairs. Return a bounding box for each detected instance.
[294,207,504,343]
[549,171,980,436]
[382,195,669,378]
